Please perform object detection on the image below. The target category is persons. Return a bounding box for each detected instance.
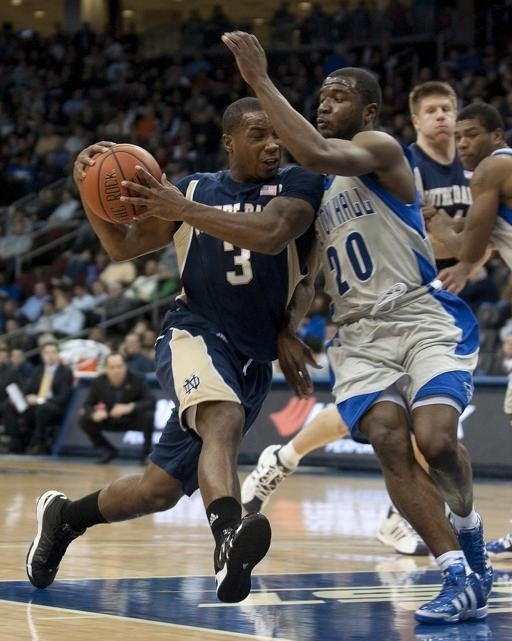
[0,0,245,454]
[212,26,494,630]
[77,356,155,465]
[24,93,324,605]
[459,251,512,374]
[267,1,512,144]
[296,292,337,352]
[235,79,477,559]
[422,96,512,566]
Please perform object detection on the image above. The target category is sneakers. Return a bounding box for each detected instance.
[239,444,290,515]
[377,508,512,624]
[28,489,86,588]
[214,511,270,603]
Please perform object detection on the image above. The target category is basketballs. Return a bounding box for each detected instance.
[82,143,161,223]
